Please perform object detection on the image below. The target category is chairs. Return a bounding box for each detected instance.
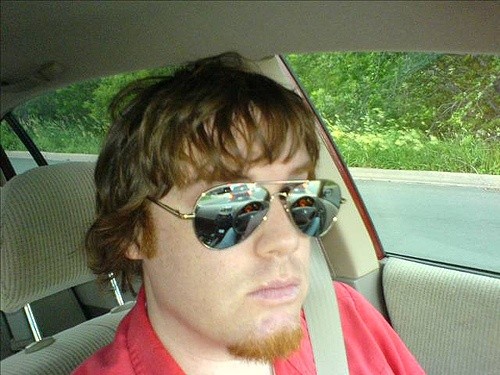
[0,162,143,375]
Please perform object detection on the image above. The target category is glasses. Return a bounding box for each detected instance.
[146,178,347,251]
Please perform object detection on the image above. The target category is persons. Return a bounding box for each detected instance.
[68,51,428,375]
[213,227,238,249]
[303,217,320,236]
